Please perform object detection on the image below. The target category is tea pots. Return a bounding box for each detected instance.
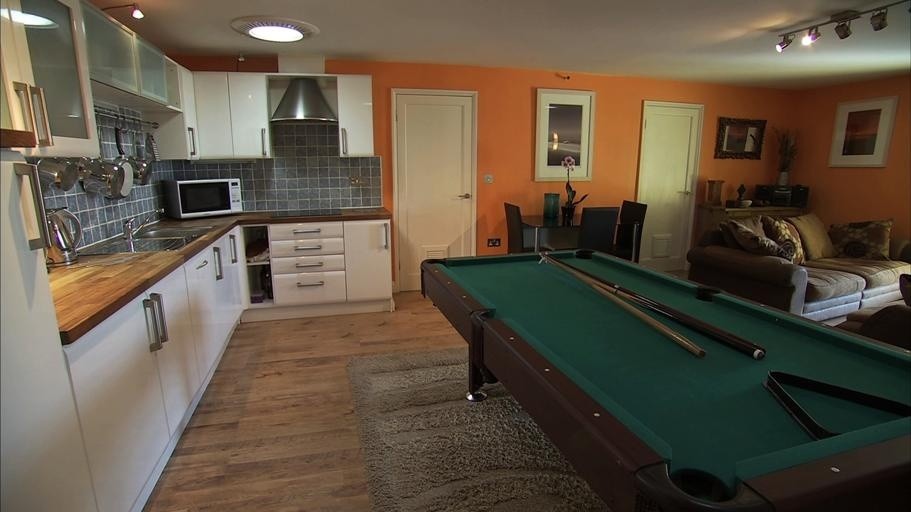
[44,208,83,267]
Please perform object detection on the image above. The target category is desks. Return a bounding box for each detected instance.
[419,246,911,511]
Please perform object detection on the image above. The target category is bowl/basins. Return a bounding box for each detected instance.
[736,200,752,207]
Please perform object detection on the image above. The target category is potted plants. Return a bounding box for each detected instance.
[777,130,800,185]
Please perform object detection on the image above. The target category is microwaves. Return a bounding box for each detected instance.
[160,178,244,219]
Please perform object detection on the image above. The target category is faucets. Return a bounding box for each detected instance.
[122,206,166,239]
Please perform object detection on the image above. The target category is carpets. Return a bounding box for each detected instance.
[343,343,613,511]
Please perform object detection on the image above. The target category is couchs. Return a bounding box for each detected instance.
[834,274,911,351]
[687,207,911,321]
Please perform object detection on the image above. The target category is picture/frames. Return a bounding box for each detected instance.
[714,116,767,160]
[827,95,901,168]
[534,87,596,182]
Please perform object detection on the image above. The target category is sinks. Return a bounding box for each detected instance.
[79,235,199,255]
[138,225,216,241]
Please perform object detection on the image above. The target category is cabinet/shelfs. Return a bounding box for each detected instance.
[0,0,102,158]
[243,222,346,309]
[190,70,272,164]
[342,219,393,303]
[80,0,182,112]
[335,73,375,159]
[184,225,245,385]
[140,35,200,161]
[61,266,204,511]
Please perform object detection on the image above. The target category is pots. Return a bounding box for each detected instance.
[82,125,125,197]
[37,158,80,192]
[112,127,134,198]
[129,140,152,185]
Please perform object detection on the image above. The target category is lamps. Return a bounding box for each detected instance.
[774,8,887,54]
[101,2,144,20]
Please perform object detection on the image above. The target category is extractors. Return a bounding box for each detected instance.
[270,78,338,122]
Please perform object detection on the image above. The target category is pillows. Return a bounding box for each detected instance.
[718,210,894,265]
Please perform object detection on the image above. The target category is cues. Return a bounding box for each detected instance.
[545,253,766,359]
[538,252,706,358]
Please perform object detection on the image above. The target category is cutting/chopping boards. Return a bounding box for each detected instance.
[48,251,184,342]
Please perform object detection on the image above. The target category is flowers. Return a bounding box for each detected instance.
[561,157,589,208]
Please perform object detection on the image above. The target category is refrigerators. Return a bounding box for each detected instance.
[1,150,97,512]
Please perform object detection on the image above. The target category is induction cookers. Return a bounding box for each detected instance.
[271,206,342,217]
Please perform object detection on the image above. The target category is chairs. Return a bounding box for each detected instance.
[504,200,647,264]
